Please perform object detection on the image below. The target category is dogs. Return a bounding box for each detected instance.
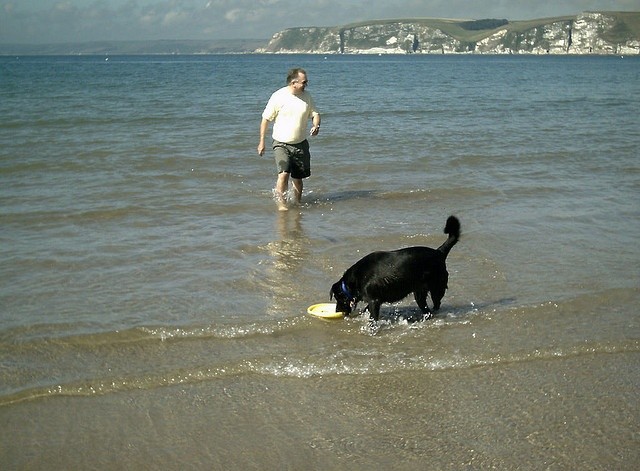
[329,215,461,325]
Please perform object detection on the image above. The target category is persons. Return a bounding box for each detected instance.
[257,68,321,212]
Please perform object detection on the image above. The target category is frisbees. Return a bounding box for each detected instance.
[307,303,344,320]
